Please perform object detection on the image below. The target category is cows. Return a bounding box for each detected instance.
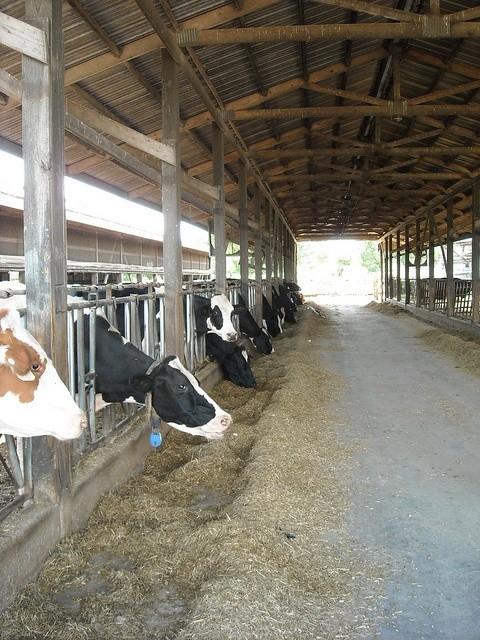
[263,294,283,338]
[290,293,302,305]
[205,334,257,387]
[272,286,286,325]
[283,282,301,293]
[1,281,232,440]
[279,284,298,324]
[0,307,88,442]
[75,285,246,359]
[228,283,275,356]
